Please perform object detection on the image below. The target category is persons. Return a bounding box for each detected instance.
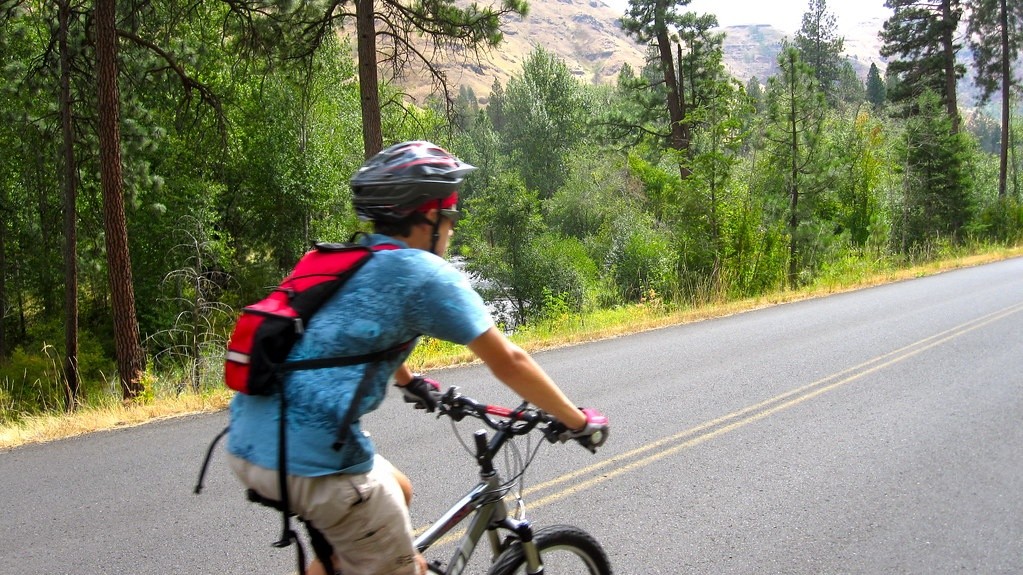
[223,139,609,575]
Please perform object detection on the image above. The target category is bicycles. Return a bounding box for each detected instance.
[244,373,616,575]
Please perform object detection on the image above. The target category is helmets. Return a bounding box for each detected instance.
[350,140,478,220]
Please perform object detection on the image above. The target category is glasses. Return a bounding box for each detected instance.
[436,208,460,226]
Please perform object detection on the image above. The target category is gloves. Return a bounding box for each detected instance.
[572,405,608,437]
[394,374,438,413]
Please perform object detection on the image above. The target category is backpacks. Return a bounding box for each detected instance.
[226,233,423,394]
